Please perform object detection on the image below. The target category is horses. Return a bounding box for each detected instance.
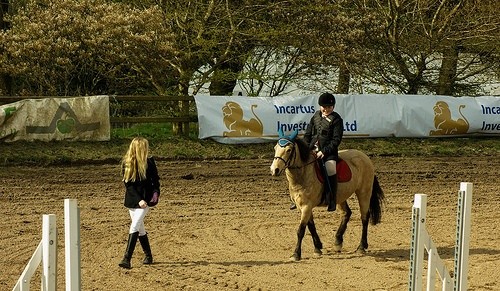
[270,129,385,262]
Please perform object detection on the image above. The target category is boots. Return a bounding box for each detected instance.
[325,172,338,212]
[118,231,139,269]
[289,202,297,210]
[139,232,153,264]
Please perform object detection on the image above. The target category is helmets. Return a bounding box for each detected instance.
[317,92,336,109]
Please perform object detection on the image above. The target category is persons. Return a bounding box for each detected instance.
[290,91,344,212]
[119,136,161,269]
